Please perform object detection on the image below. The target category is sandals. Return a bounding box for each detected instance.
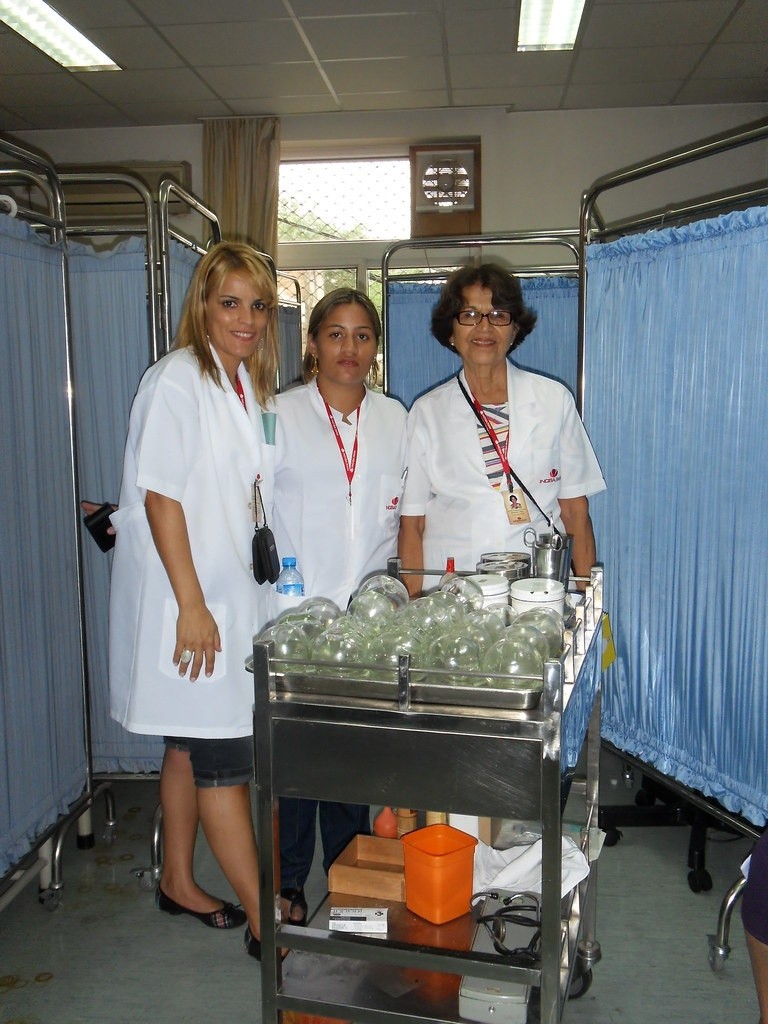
[280,887,308,926]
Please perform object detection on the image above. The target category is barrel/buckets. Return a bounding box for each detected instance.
[510,578,565,619]
[466,575,509,608]
[477,552,531,582]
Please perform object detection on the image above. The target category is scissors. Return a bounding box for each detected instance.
[523,527,564,577]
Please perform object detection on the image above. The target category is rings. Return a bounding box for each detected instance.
[181,648,192,663]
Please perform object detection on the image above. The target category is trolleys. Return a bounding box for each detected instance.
[245,564,605,1023]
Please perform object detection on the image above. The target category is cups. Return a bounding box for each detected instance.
[532,533,574,591]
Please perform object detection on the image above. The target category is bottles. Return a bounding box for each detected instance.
[398,808,416,838]
[440,557,457,594]
[275,557,303,614]
[374,808,398,839]
[426,811,446,827]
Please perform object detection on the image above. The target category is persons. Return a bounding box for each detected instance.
[82,288,408,929]
[397,262,607,599]
[108,242,289,965]
[742,828,768,1024]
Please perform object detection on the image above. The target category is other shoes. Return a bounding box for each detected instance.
[155,880,248,929]
[244,927,285,965]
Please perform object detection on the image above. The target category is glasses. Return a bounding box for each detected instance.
[452,310,513,326]
